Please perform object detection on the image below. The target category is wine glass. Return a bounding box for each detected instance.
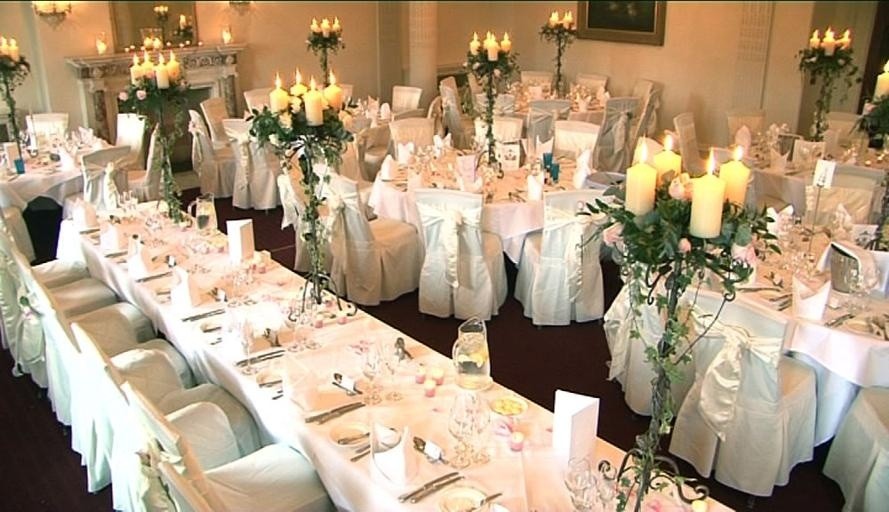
[761,208,872,314]
[118,188,320,351]
[361,335,404,405]
[22,125,93,162]
[563,456,618,512]
[411,144,543,196]
[445,391,491,469]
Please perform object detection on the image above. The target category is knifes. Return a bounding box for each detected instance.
[304,401,365,426]
[350,443,369,463]
[234,349,287,368]
[397,471,467,506]
[465,492,504,512]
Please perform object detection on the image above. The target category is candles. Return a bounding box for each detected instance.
[0,45,9,56]
[142,63,155,80]
[9,46,20,62]
[309,15,343,42]
[823,37,835,57]
[840,36,852,51]
[166,62,180,82]
[130,65,144,84]
[470,28,511,63]
[153,66,169,89]
[151,5,169,15]
[548,10,573,29]
[810,38,821,50]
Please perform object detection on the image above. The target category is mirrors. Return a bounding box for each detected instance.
[107,0,198,48]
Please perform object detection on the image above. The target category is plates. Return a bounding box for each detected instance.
[435,484,490,512]
[329,421,370,448]
[487,396,528,417]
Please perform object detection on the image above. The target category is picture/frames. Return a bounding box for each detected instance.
[575,0,666,46]
